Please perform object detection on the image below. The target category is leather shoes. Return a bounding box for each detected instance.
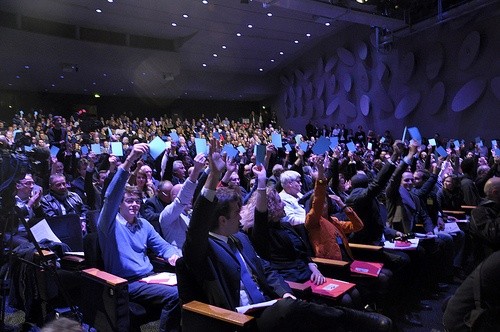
[406,302,432,311]
[394,312,424,328]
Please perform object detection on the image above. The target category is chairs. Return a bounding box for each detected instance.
[27,203,478,332]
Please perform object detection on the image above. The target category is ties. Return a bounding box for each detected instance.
[227,237,266,304]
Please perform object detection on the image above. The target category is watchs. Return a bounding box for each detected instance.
[308,263,318,267]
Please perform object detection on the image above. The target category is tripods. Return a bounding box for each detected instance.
[0,195,81,325]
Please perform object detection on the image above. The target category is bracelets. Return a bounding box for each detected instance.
[126,156,134,163]
[342,204,347,210]
[257,187,267,191]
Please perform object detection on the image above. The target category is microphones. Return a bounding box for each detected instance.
[395,232,415,240]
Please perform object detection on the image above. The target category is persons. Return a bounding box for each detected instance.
[1,107,500,332]
